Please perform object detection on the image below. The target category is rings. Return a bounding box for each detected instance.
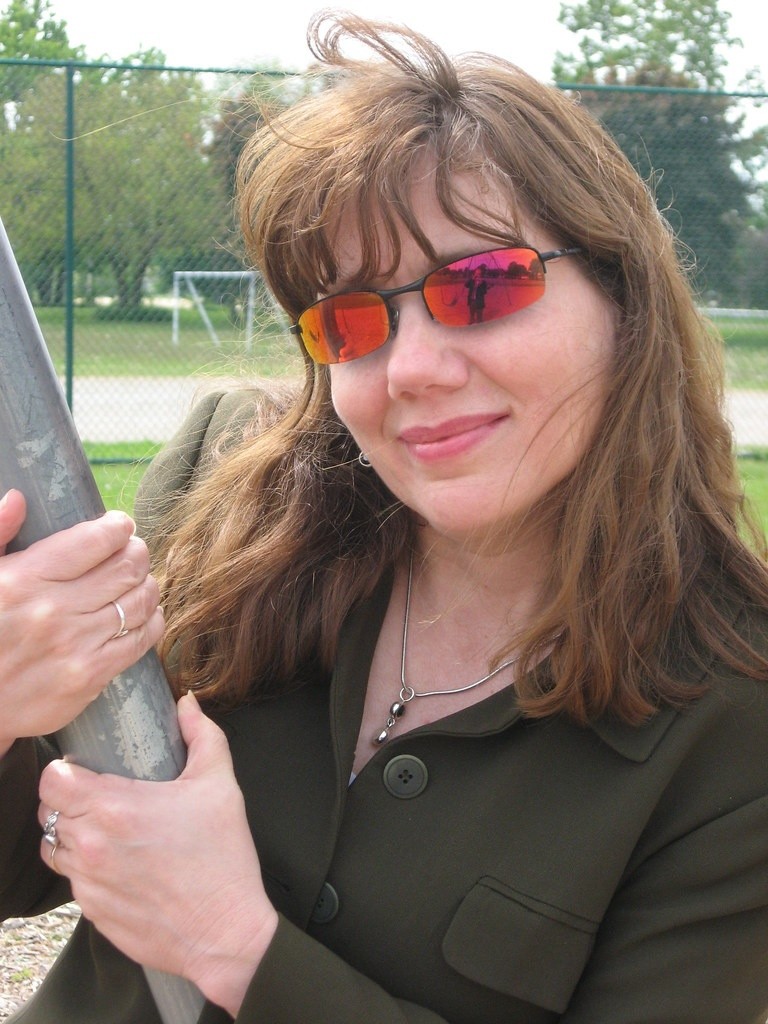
[111,600,131,640]
[50,848,62,877]
[42,811,60,847]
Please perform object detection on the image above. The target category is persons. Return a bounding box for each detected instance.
[465,267,487,324]
[1,14,767,1024]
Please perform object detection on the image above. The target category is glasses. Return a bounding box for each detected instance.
[288,247,586,365]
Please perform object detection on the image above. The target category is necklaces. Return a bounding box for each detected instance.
[370,525,567,745]
